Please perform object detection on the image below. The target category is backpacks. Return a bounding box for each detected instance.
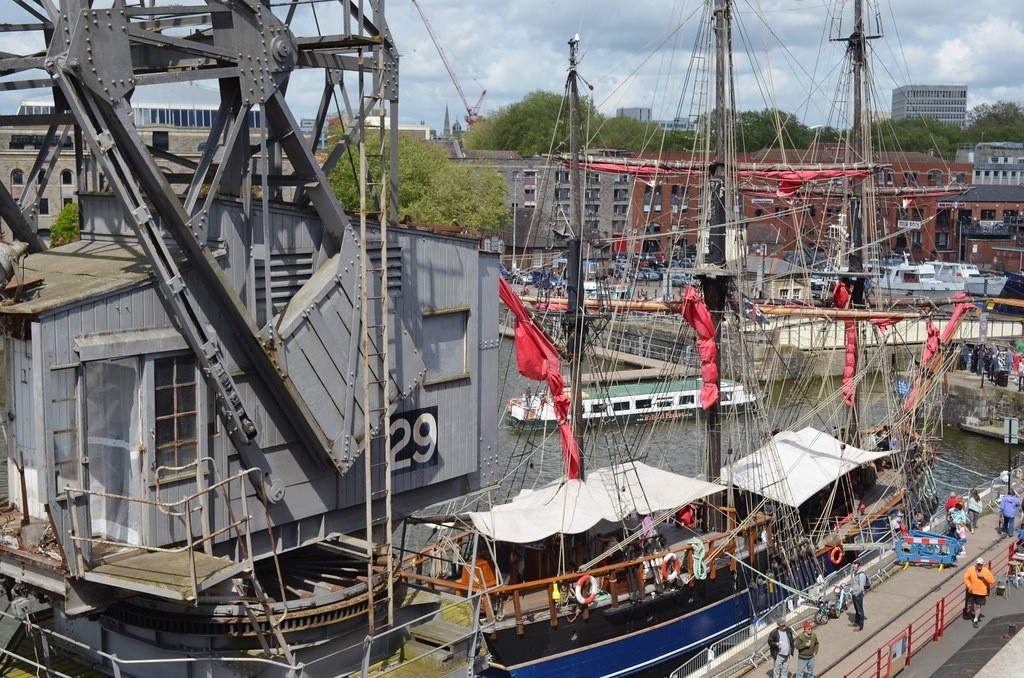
[855,570,871,589]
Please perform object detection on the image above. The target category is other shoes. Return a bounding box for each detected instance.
[971,528,974,534]
[972,614,975,622]
[973,621,978,628]
[848,622,858,627]
[959,549,964,552]
[853,626,863,632]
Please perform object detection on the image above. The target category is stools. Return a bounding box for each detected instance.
[1012,551,1024,569]
[1007,560,1024,589]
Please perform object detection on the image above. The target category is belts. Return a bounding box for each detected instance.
[779,655,789,658]
[799,656,813,660]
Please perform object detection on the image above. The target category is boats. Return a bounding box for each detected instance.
[863,259,964,301]
[923,259,1008,297]
[412,1,946,678]
[501,374,768,431]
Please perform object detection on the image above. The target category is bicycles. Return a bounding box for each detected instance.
[815,580,850,624]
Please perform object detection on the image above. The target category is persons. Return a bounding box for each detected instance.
[943,487,967,557]
[767,618,794,678]
[793,622,820,678]
[963,558,995,627]
[968,489,982,533]
[536,272,683,306]
[954,334,1024,389]
[1000,488,1020,537]
[849,561,865,633]
[597,535,624,567]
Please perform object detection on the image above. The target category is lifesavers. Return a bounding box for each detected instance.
[662,553,680,580]
[576,575,598,604]
[831,547,842,564]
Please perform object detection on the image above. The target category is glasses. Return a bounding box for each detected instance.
[806,627,812,630]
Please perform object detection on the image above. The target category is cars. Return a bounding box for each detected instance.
[496,249,909,308]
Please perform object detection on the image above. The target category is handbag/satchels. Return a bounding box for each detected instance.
[998,514,1004,529]
[949,521,957,528]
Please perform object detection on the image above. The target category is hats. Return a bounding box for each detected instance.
[776,619,787,625]
[803,621,810,628]
[976,558,984,564]
